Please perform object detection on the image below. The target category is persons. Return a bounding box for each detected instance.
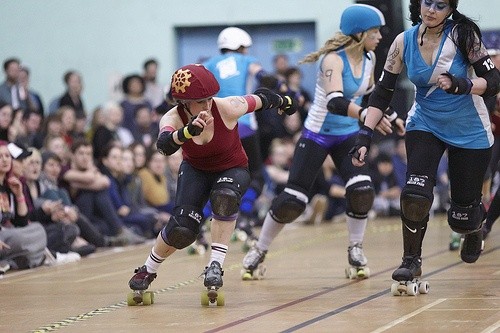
[352,0,500,297]
[127,64,298,306]
[0,54,500,274]
[241,4,405,280]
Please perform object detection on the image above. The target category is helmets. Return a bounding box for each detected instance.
[217,27,253,52]
[170,64,221,100]
[339,4,386,36]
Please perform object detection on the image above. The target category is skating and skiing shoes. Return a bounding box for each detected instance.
[392,255,429,297]
[241,244,267,281]
[199,260,226,306]
[345,242,370,279]
[459,228,486,263]
[127,264,157,307]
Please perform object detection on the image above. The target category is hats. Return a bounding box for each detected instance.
[8,144,32,160]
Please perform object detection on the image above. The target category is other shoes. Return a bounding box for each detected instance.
[44,227,145,266]
